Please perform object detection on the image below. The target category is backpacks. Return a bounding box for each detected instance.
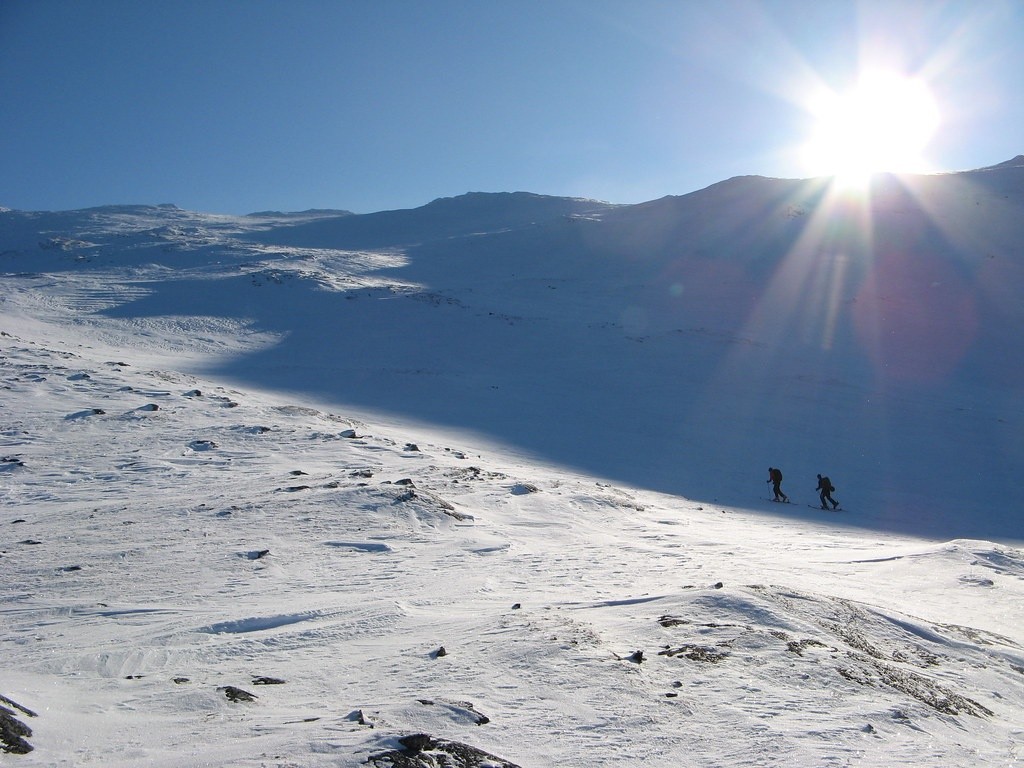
[823,477,831,490]
[773,469,782,481]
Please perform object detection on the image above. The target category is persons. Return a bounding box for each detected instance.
[767,468,787,502]
[816,474,840,511]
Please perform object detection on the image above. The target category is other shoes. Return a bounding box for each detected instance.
[783,497,787,502]
[773,498,779,501]
[833,502,838,508]
[820,505,828,509]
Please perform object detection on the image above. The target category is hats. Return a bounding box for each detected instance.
[768,468,772,471]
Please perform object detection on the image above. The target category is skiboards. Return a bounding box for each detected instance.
[808,504,848,512]
[768,498,798,505]
